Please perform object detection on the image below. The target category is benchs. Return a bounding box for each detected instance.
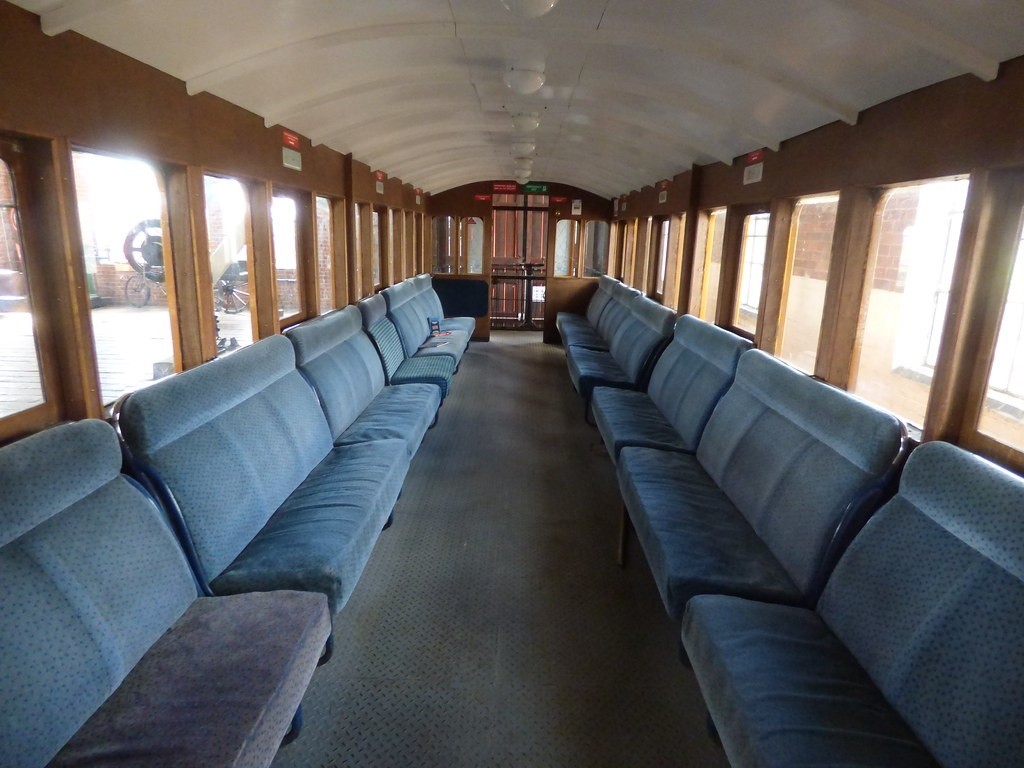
[410,273,476,354]
[555,274,621,332]
[566,294,677,428]
[358,292,456,429]
[379,281,470,376]
[0,413,335,768]
[610,347,904,618]
[115,331,411,668]
[680,438,1024,768]
[588,313,759,472]
[560,282,644,353]
[287,304,442,501]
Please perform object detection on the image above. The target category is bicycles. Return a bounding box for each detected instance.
[123,253,251,356]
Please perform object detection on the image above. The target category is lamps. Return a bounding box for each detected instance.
[501,0,559,19]
[513,157,534,169]
[497,68,546,96]
[509,114,540,133]
[516,177,530,185]
[514,169,532,178]
[510,142,536,156]
